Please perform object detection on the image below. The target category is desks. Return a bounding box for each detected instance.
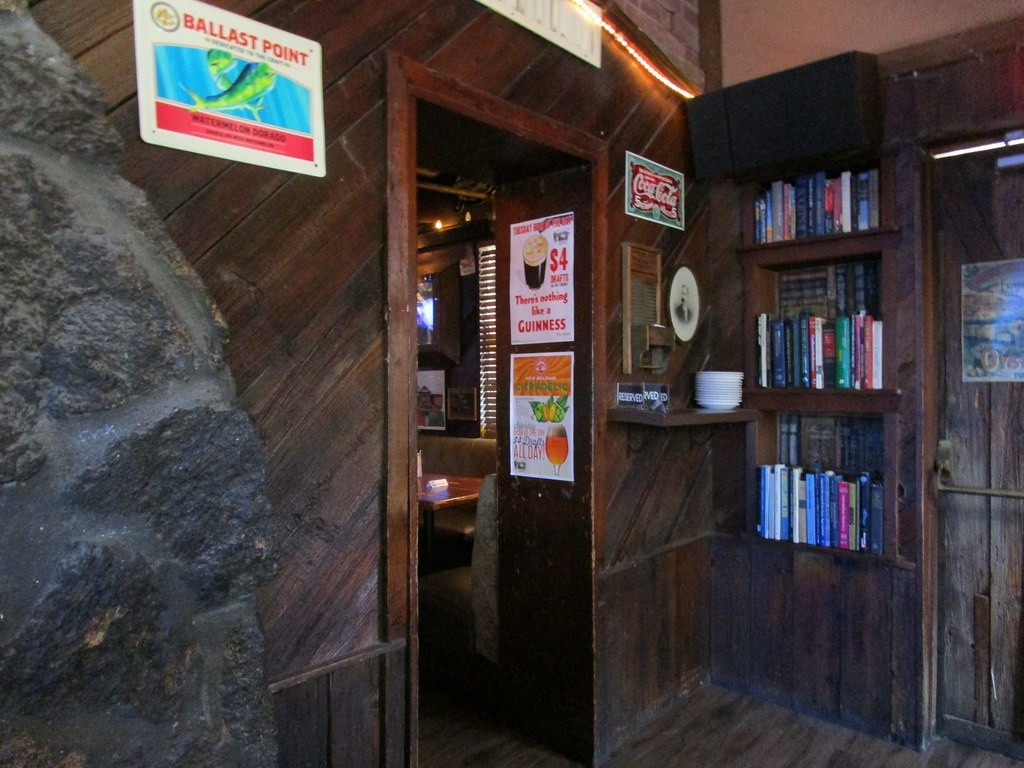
[418,472,486,572]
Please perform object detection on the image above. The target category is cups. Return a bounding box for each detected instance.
[522,234,548,290]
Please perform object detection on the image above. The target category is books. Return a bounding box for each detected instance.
[756,261,883,389]
[754,167,880,244]
[759,412,886,555]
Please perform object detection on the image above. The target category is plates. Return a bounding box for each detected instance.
[694,371,745,410]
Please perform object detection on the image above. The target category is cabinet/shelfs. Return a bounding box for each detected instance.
[745,151,901,565]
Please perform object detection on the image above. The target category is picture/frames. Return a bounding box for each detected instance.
[448,387,478,422]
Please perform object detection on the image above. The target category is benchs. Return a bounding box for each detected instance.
[418,436,497,540]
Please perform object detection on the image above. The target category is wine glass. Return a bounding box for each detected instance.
[544,423,569,476]
